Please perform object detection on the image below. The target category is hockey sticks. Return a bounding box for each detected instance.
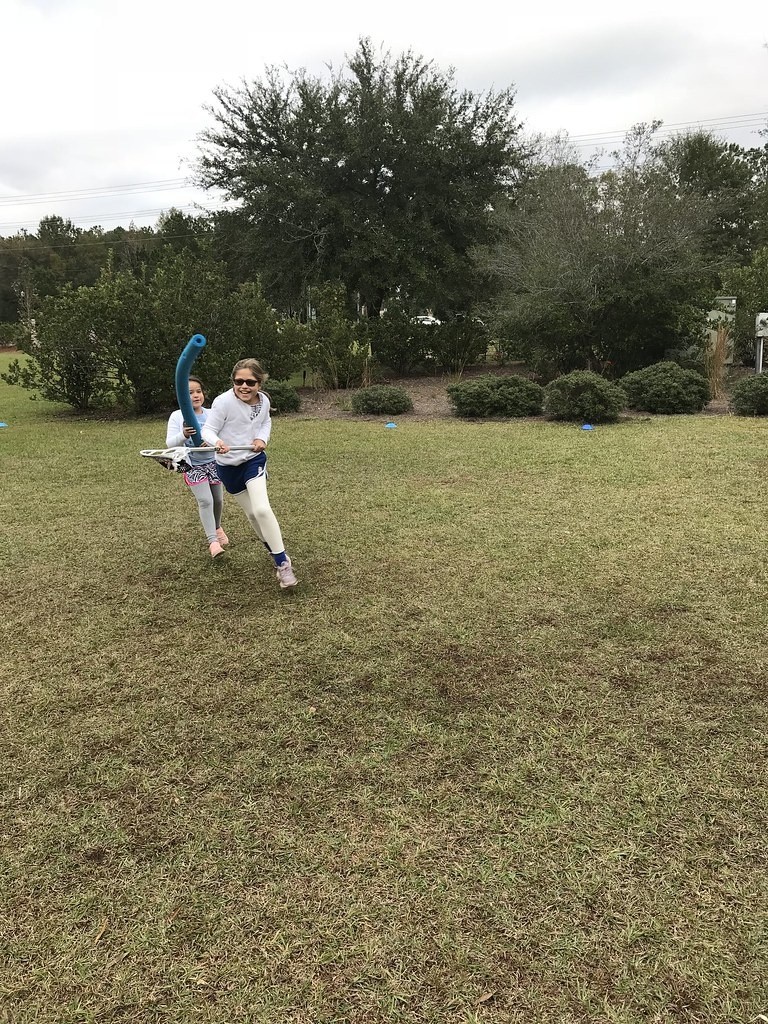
[139,444,255,473]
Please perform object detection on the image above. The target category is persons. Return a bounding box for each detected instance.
[201,358,298,588]
[166,376,228,558]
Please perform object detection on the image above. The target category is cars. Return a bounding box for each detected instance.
[410,316,447,325]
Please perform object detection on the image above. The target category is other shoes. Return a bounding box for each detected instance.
[208,540,226,558]
[215,526,228,546]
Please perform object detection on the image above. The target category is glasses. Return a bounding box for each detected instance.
[232,378,261,387]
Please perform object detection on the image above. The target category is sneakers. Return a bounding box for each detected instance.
[274,553,298,588]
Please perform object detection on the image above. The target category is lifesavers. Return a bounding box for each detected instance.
[173,333,207,448]
